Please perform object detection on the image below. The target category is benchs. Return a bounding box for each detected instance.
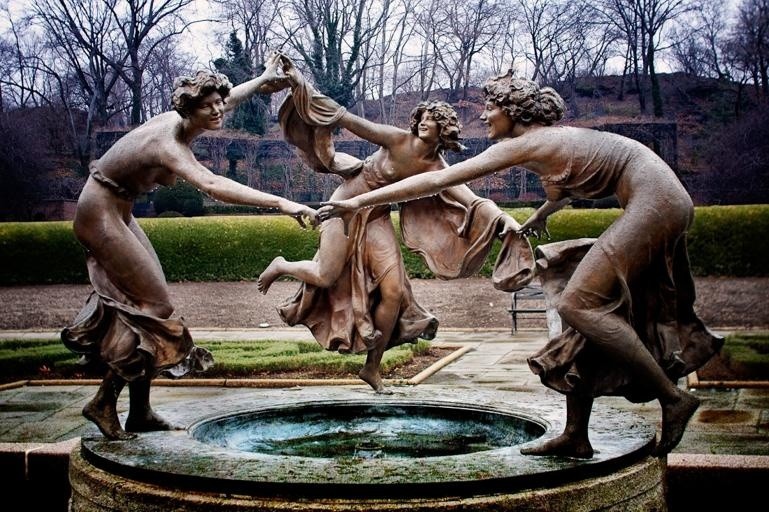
[507,284,547,337]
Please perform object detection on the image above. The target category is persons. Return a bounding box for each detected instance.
[61,51,324,441]
[257,53,539,394]
[314,71,723,458]
